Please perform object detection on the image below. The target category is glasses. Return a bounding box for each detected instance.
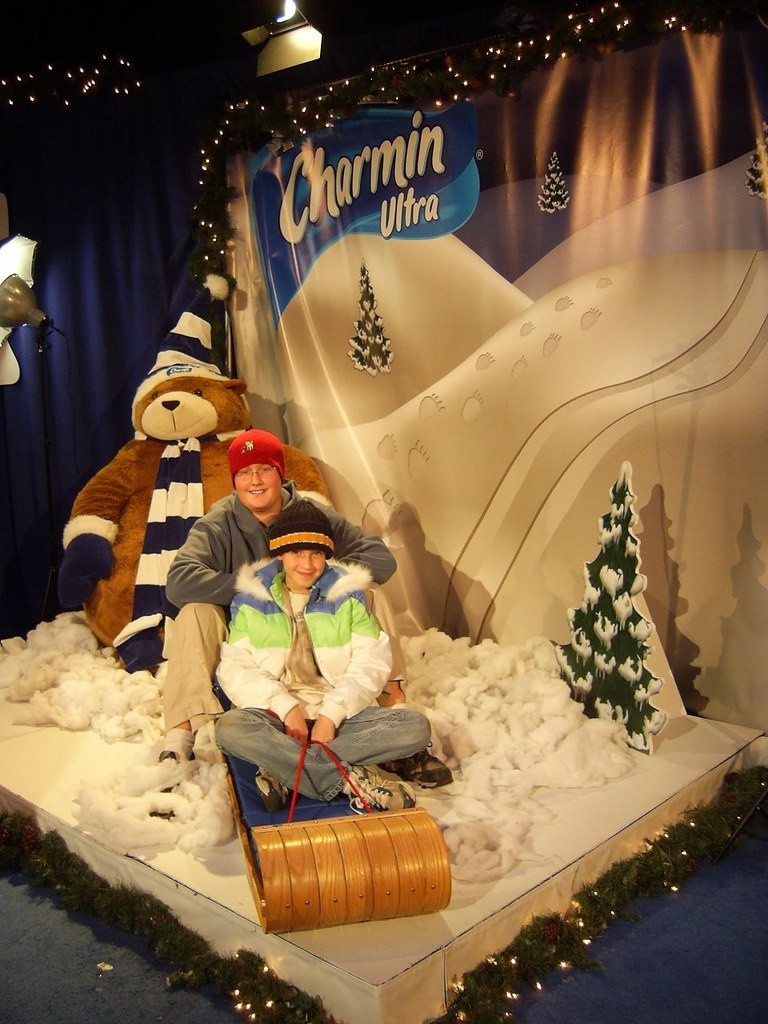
[237,467,276,478]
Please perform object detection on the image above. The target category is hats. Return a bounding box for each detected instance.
[270,499,335,559]
[228,429,285,488]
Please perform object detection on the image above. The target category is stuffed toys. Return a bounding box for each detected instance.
[60,275,332,675]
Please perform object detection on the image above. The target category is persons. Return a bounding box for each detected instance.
[149,429,453,819]
[216,501,431,810]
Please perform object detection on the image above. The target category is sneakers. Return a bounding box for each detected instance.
[255,767,289,814]
[348,765,416,814]
[149,751,197,821]
[401,741,453,787]
